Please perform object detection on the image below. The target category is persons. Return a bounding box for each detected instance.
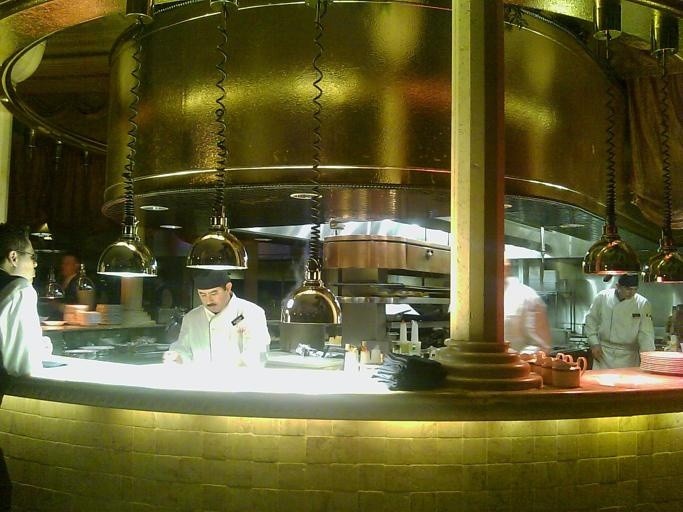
[503,251,551,356]
[163,271,270,368]
[60,254,85,308]
[0,220,54,512]
[148,258,178,309]
[585,275,656,369]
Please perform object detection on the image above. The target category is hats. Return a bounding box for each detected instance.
[618,273,638,287]
[192,269,229,290]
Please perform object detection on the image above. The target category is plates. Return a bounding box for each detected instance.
[42,304,121,327]
[640,352,683,374]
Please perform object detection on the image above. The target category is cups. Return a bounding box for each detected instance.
[525,352,587,388]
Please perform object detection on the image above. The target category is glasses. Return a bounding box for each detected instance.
[14,249,39,262]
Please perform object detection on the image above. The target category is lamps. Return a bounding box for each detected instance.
[280,0,343,325]
[643,9,683,284]
[30,1,158,300]
[581,0,642,274]
[185,0,249,271]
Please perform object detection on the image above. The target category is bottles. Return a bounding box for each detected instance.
[344,340,381,373]
[398,320,421,355]
